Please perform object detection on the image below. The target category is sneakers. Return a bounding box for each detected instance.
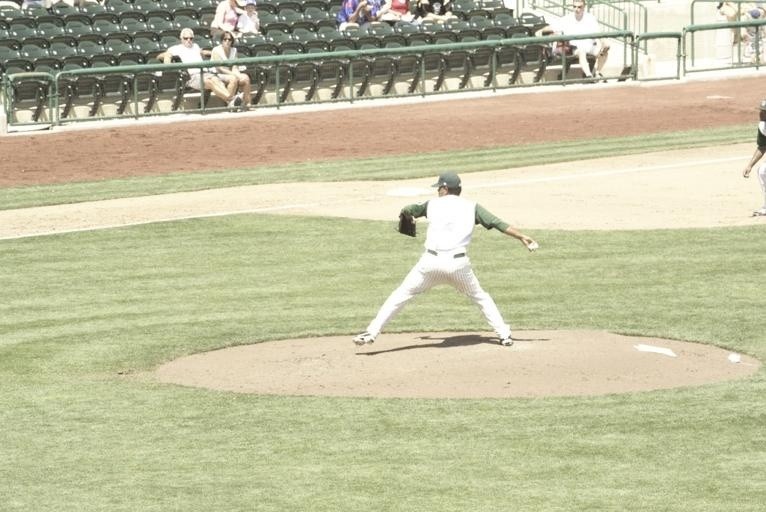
[500,336,513,345]
[352,332,375,345]
[227,92,257,112]
[586,72,608,83]
[753,208,766,217]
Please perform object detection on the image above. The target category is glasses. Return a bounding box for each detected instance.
[223,38,232,41]
[183,36,194,40]
[573,5,582,8]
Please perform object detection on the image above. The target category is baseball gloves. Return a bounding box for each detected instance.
[399,215,415,237]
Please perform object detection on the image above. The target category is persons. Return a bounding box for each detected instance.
[164,28,254,111]
[352,171,537,345]
[743,100,766,216]
[210,0,260,37]
[336,0,455,31]
[535,0,610,83]
[717,1,766,64]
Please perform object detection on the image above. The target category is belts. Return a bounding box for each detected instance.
[428,249,465,258]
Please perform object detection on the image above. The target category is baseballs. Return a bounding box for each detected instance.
[529,242,538,250]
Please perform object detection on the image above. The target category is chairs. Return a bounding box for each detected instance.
[1,0,605,104]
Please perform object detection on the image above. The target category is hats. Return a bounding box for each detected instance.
[235,0,256,7]
[432,172,461,187]
[760,99,766,110]
